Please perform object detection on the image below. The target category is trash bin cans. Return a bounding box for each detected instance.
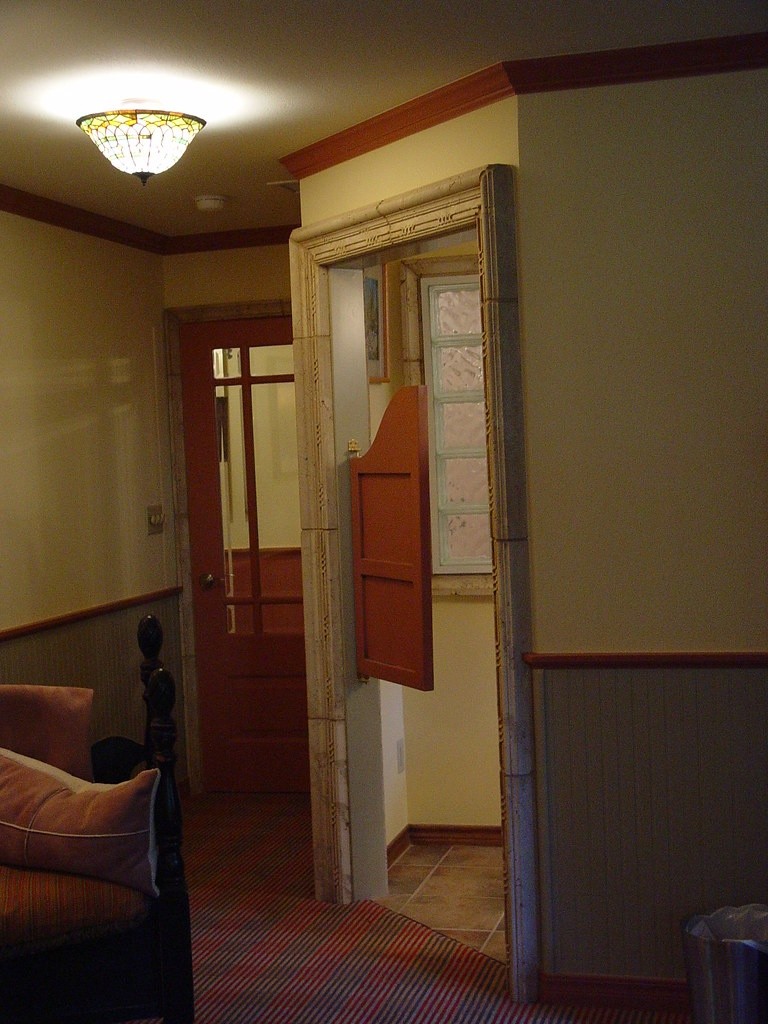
[678,907,768,1024]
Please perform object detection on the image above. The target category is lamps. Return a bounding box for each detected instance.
[75,109,207,188]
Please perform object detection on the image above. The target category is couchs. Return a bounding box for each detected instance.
[0,737,165,1024]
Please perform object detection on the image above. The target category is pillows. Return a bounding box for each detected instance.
[0,684,96,781]
[1,750,160,900]
